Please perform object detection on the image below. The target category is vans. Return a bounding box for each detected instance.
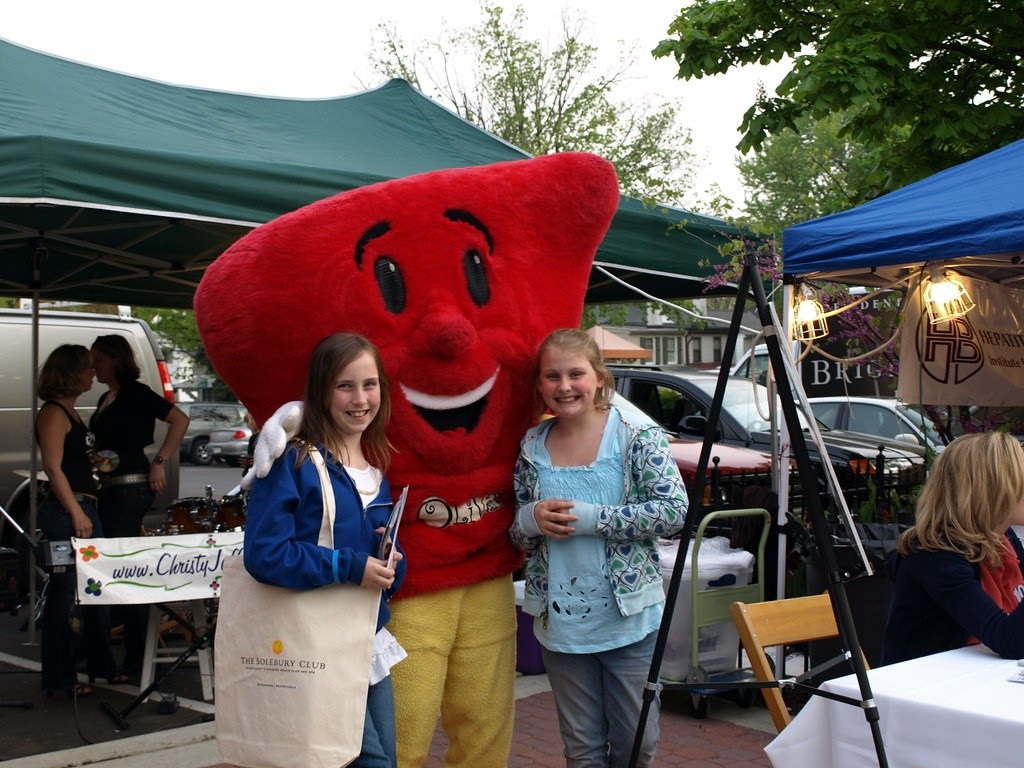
[174,401,248,466]
[0,307,180,546]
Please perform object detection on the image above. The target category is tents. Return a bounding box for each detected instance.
[775,141,1024,688]
[0,37,780,495]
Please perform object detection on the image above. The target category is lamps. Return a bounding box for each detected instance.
[924,260,977,324]
[793,280,830,341]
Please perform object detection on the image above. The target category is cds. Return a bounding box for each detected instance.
[94,450,120,472]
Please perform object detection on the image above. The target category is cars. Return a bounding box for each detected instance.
[206,418,252,466]
[602,286,1024,526]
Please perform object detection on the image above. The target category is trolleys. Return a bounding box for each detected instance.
[657,508,777,720]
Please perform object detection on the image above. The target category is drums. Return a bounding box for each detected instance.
[165,497,218,534]
[214,494,251,532]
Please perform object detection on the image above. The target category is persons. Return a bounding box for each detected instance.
[510,329,690,768]
[881,433,1024,666]
[35,335,192,697]
[212,332,406,768]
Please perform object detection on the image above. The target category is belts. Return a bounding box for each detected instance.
[47,492,98,509]
[98,474,149,488]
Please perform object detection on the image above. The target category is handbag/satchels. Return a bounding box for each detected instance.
[212,436,383,768]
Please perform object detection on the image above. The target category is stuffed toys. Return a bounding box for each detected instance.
[195,153,621,768]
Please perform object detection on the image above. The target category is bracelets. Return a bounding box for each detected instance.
[332,549,339,585]
[154,455,168,465]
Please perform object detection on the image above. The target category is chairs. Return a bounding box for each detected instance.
[730,591,871,734]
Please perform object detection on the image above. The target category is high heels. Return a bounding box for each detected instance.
[46,683,92,698]
[89,672,124,685]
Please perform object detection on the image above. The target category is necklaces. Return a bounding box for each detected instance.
[105,389,117,406]
[65,402,77,418]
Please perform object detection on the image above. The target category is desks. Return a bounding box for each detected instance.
[763,641,1024,768]
